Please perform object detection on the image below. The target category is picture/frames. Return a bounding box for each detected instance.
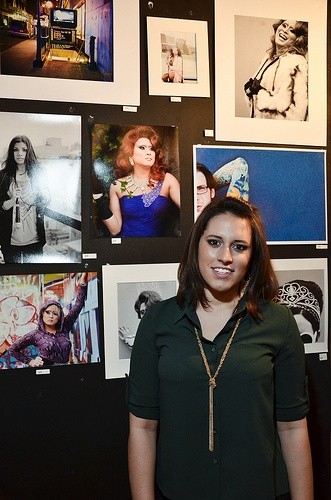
[215,1,329,148]
[147,16,210,99]
[0,1,142,107]
[102,262,183,380]
[269,258,329,353]
[192,144,328,246]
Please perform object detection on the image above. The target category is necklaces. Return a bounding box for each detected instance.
[194,318,243,450]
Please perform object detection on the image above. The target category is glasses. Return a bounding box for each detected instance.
[45,310,58,315]
[197,185,208,195]
[300,331,317,343]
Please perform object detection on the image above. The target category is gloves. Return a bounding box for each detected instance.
[95,180,113,220]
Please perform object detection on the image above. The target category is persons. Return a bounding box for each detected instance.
[167,47,183,83]
[9,272,88,367]
[270,280,324,343]
[195,157,250,218]
[0,135,52,263]
[244,18,309,121]
[92,124,180,237]
[118,291,162,349]
[126,196,313,500]
